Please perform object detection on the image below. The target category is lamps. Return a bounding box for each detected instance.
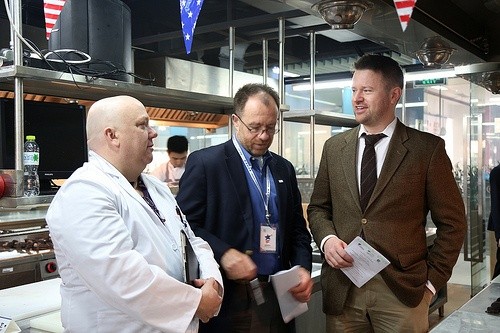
[320,5,364,31]
[417,50,450,69]
[483,81,500,94]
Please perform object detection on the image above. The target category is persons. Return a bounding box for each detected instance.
[151,135,192,186]
[301,53,467,333]
[47,95,227,333]
[173,81,312,333]
[484,161,500,314]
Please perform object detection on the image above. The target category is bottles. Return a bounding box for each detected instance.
[22,135,41,197]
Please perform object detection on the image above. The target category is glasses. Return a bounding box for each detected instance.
[233,112,280,137]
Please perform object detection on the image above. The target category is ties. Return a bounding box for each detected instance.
[359,133,387,214]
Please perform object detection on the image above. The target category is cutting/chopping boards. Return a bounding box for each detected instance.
[0,278,67,323]
[29,310,67,333]
